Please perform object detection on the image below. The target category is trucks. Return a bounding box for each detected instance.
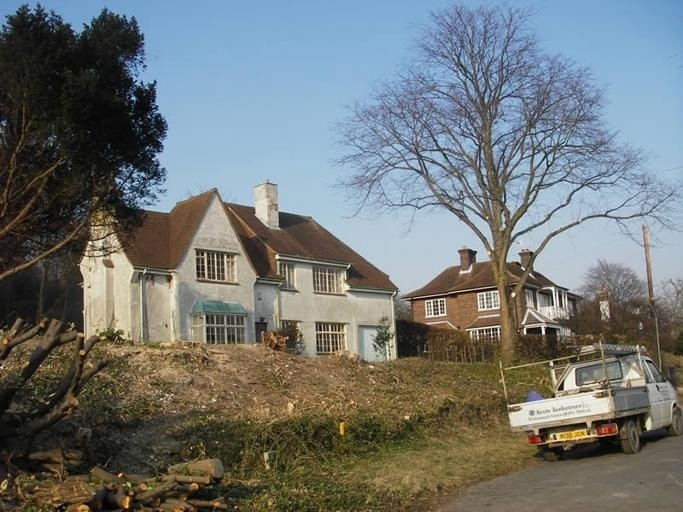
[497,343,682,456]
